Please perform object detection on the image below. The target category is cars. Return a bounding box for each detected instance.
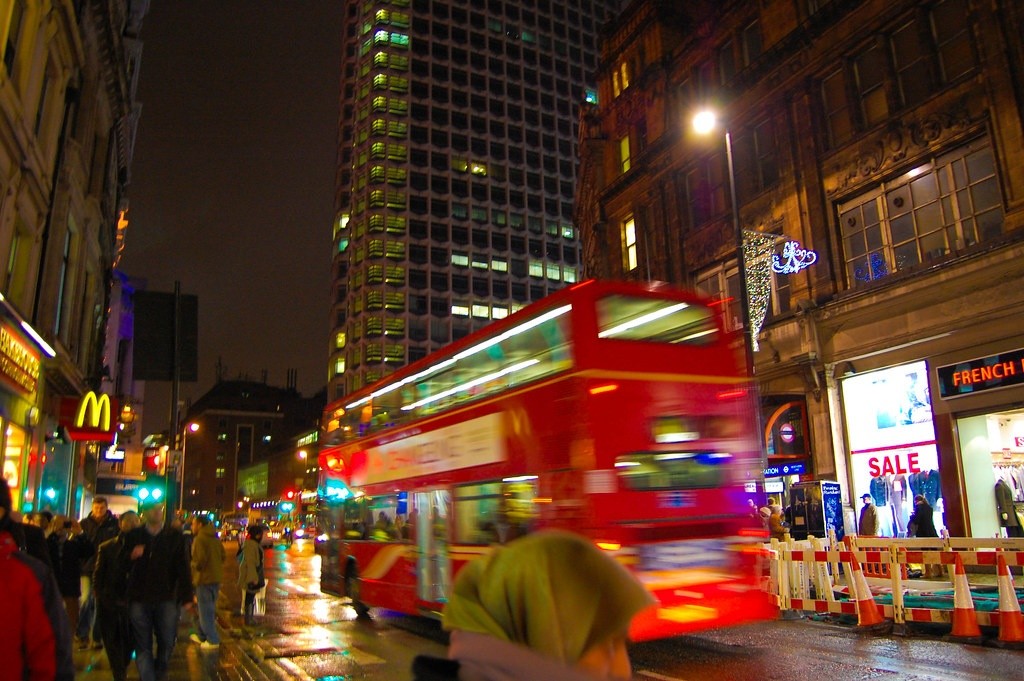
[221,522,274,550]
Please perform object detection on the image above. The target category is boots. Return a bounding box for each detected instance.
[245,603,259,626]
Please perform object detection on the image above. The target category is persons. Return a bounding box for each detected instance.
[285,519,295,545]
[995,475,1024,551]
[375,510,398,540]
[869,467,943,538]
[442,529,657,681]
[911,494,942,579]
[747,498,758,518]
[859,494,878,573]
[0,475,265,681]
[760,497,789,541]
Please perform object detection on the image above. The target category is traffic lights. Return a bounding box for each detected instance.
[288,490,295,499]
[280,502,295,512]
[138,487,165,501]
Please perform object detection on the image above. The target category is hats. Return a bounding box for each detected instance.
[769,505,783,512]
[860,494,870,498]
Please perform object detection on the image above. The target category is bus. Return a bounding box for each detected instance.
[314,277,780,644]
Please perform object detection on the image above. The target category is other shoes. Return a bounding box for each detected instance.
[92,640,103,650]
[200,640,220,649]
[78,640,88,651]
[190,633,203,643]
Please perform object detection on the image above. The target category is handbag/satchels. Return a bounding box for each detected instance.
[249,567,265,590]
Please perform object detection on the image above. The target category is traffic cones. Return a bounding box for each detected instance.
[849,551,892,633]
[942,553,986,643]
[984,548,1024,650]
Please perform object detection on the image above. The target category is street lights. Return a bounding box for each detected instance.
[694,108,758,405]
[180,423,200,510]
